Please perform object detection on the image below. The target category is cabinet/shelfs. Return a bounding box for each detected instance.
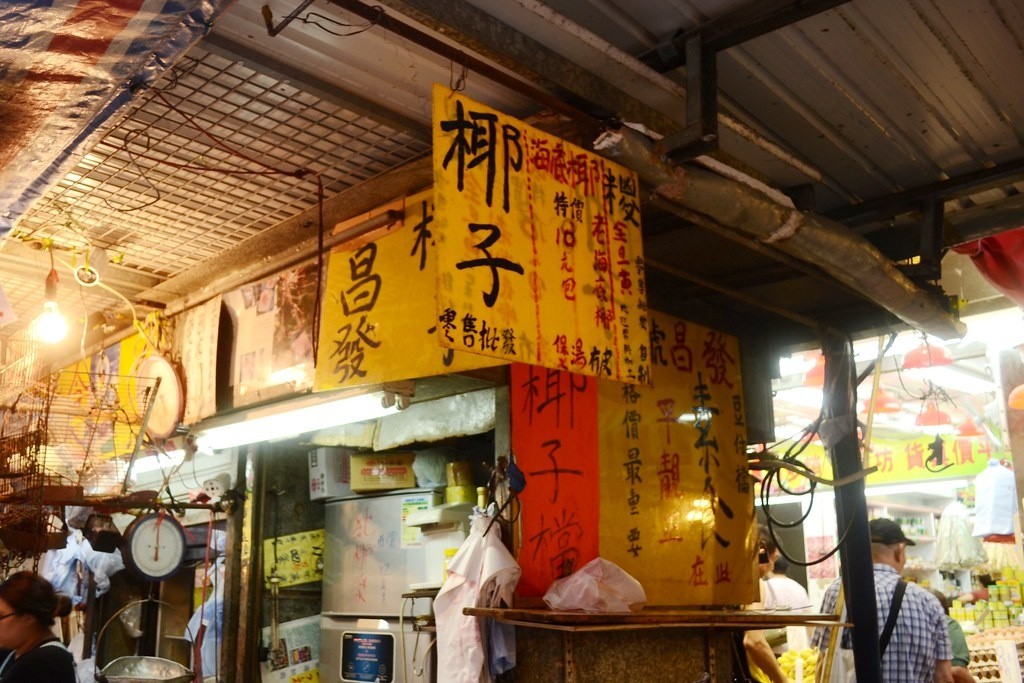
[864,500,974,597]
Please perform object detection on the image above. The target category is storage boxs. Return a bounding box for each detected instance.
[349,452,416,491]
[307,447,360,501]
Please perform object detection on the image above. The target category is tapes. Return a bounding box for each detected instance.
[443,486,476,504]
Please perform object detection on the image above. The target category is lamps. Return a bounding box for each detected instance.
[191,380,418,457]
[36,271,69,343]
[805,336,987,438]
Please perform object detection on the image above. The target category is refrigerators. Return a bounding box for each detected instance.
[318,489,443,683]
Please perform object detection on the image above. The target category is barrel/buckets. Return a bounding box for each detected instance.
[92,594,198,683]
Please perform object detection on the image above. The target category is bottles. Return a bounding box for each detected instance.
[477,485,487,512]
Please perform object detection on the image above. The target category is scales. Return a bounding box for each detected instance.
[93,511,196,683]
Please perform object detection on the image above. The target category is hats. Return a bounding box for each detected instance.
[869,517,916,546]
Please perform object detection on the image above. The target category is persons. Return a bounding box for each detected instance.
[958,574,998,605]
[0,570,76,683]
[922,586,976,683]
[810,516,953,683]
[743,525,789,683]
[763,554,810,658]
[277,290,311,365]
